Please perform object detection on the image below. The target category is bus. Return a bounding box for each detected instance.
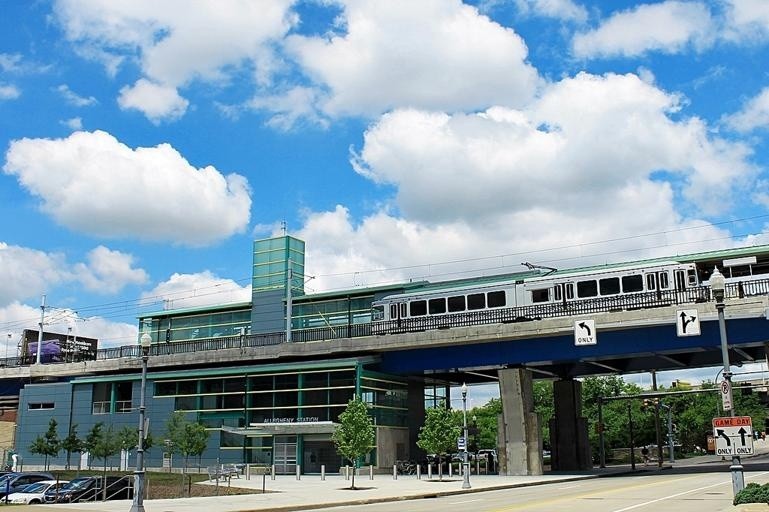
[368,260,701,335]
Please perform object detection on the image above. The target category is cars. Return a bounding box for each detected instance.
[1,470,135,505]
[542,452,551,463]
[427,449,498,467]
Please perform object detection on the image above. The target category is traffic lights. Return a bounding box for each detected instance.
[654,398,660,406]
[644,399,649,409]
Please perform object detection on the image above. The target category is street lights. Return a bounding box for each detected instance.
[130,332,153,512]
[707,264,743,502]
[472,415,477,470]
[460,382,471,489]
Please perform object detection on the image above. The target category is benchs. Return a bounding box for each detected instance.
[207,465,242,482]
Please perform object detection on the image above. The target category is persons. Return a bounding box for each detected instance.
[761,430,765,441]
[754,430,758,440]
[11,452,18,472]
[309,452,317,473]
[642,447,649,467]
[434,453,441,473]
[422,465,427,473]
[488,452,494,473]
[423,457,429,474]
